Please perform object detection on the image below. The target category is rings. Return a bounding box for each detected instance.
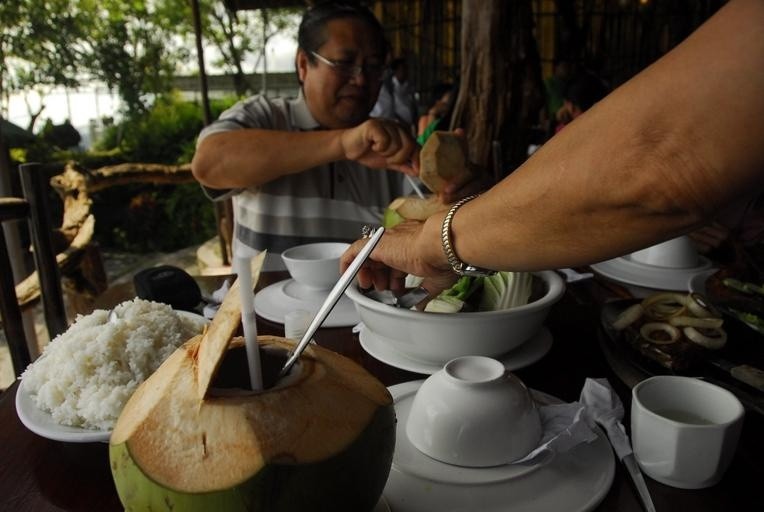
[359,222,376,237]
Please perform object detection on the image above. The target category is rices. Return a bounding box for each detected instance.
[16,297,203,433]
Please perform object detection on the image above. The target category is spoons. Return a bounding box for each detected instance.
[582,375,658,511]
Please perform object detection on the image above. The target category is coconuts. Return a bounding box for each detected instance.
[109,332,396,511]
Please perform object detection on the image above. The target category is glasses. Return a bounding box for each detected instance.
[310,49,394,81]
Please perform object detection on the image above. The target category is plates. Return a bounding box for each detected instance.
[380,378,615,512]
[358,327,556,376]
[686,269,764,340]
[587,254,714,291]
[254,277,361,328]
[15,310,212,444]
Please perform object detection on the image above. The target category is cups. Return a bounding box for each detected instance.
[630,376,745,491]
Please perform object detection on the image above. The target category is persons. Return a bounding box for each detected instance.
[540,53,576,118]
[190,1,496,280]
[374,44,469,148]
[688,198,763,278]
[338,1,763,311]
[550,77,605,137]
[418,83,460,148]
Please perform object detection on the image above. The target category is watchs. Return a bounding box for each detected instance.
[441,193,498,280]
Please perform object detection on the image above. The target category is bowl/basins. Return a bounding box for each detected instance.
[630,234,697,268]
[345,270,568,366]
[406,354,544,469]
[281,242,352,291]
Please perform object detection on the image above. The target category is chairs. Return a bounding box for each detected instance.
[0,161,69,391]
